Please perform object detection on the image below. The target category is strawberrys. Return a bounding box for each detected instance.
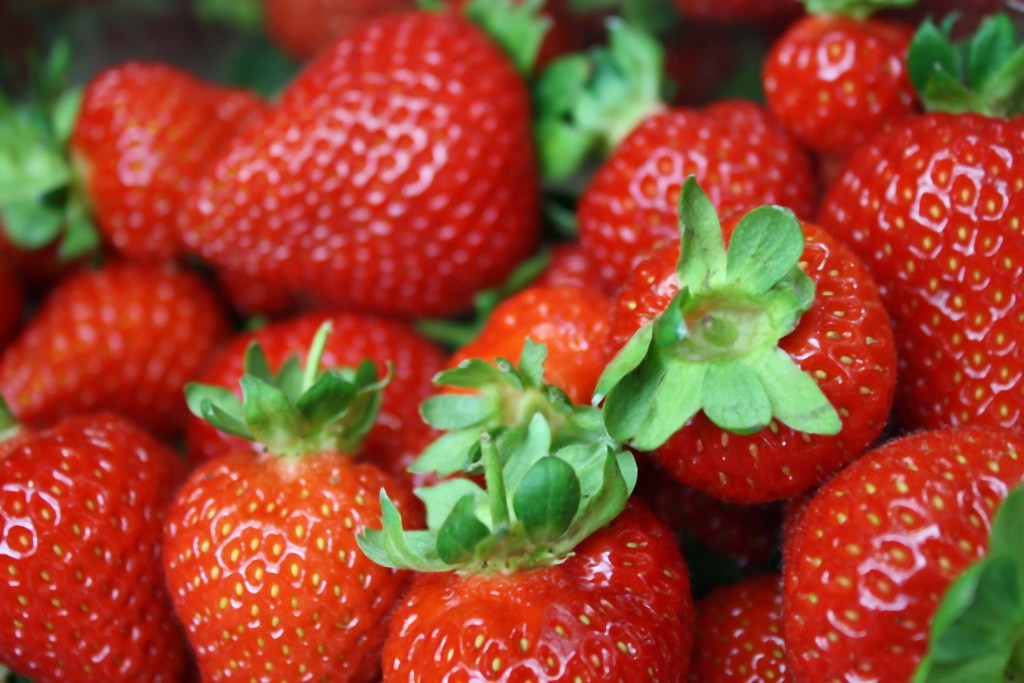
[0,0,1024,683]
